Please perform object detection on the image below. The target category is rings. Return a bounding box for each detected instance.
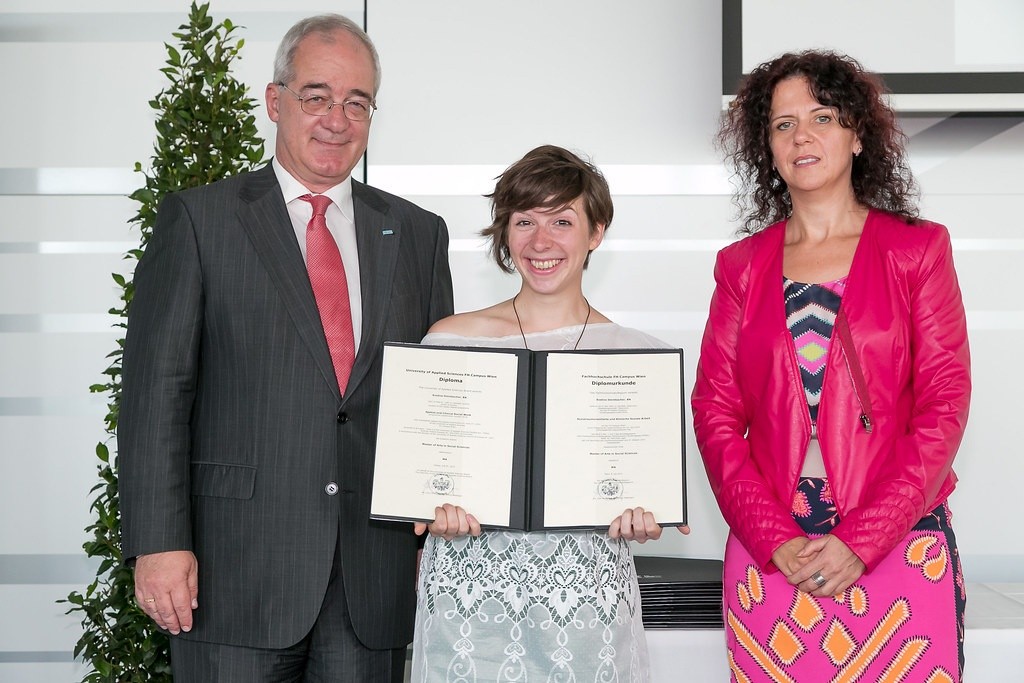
[812,571,827,587]
[144,598,154,602]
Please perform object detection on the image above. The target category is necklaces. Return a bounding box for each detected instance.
[513,292,591,350]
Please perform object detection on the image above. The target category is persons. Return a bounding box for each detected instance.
[691,49,973,683]
[409,144,690,683]
[121,14,454,683]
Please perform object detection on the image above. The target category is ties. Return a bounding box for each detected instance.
[297,193,356,397]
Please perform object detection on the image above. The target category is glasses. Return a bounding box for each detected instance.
[278,80,377,122]
